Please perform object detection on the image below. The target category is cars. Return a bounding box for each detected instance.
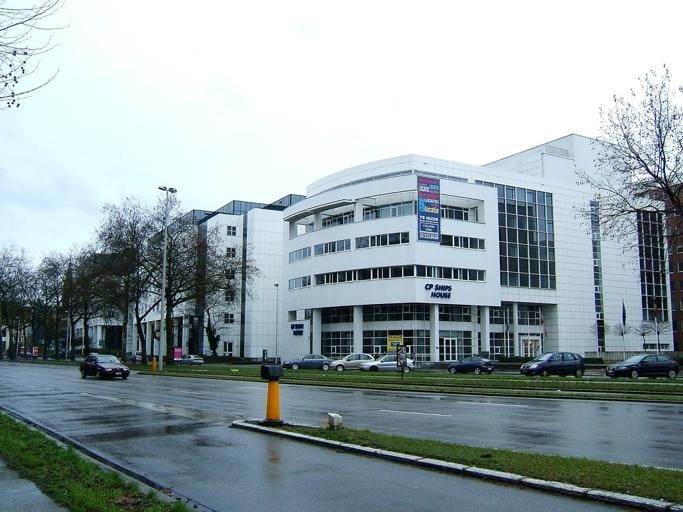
[131,351,142,363]
[178,355,204,366]
[283,353,333,371]
[605,354,678,378]
[448,355,495,376]
[359,354,416,374]
[18,347,31,356]
[79,353,130,380]
[328,353,376,371]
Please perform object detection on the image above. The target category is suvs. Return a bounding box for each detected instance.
[520,352,587,378]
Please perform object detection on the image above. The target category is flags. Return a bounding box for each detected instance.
[622,302,626,326]
[540,311,547,339]
[653,299,658,317]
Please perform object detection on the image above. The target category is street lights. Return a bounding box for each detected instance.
[64,310,70,361]
[273,283,278,364]
[156,184,178,372]
[13,317,20,359]
